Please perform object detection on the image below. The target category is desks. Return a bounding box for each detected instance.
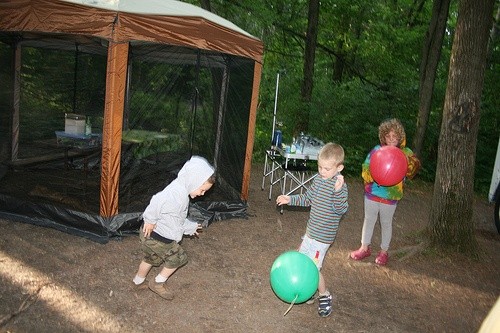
[263,143,318,214]
[27,129,166,208]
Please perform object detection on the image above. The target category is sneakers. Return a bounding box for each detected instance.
[317,291,333,318]
[306,292,318,305]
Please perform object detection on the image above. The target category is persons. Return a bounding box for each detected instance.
[276,142,349,317]
[129,156,215,301]
[351,121,420,266]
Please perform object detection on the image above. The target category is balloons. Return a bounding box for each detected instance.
[371,147,408,187]
[271,251,320,315]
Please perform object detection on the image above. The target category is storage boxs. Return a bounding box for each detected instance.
[54,130,101,149]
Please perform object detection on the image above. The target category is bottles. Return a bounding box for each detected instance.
[291,136,297,152]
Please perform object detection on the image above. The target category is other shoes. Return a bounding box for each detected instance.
[147,277,175,300]
[129,278,151,292]
[374,251,389,265]
[350,248,371,261]
